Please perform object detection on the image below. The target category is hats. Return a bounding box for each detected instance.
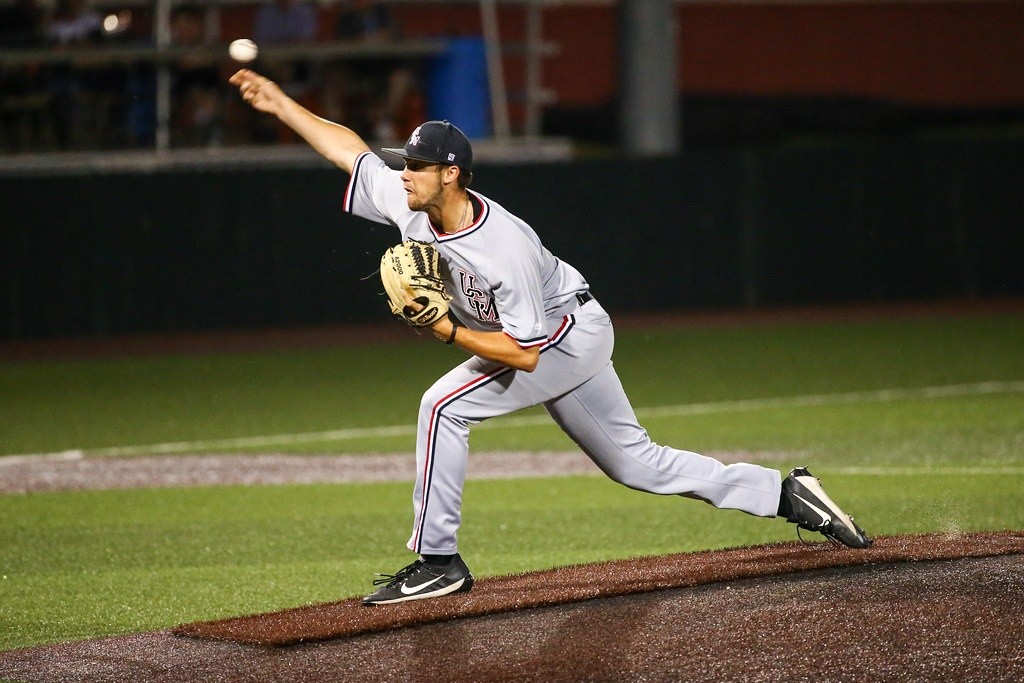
[381,119,472,170]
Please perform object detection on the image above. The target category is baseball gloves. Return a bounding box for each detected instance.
[360,235,457,337]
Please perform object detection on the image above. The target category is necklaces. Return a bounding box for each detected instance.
[454,199,470,233]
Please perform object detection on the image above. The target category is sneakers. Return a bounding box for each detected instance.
[781,466,874,549]
[362,552,474,605]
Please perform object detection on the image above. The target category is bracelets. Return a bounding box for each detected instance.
[446,324,458,345]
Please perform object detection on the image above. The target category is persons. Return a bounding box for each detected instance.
[230,68,870,606]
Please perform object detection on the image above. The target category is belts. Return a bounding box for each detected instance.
[575,290,594,307]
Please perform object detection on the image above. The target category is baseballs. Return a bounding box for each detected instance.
[229,39,258,62]
